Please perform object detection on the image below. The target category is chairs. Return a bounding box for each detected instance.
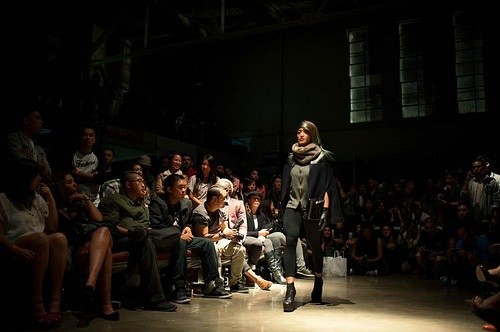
[108,247,201,303]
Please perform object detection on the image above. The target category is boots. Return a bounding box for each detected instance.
[263,247,289,285]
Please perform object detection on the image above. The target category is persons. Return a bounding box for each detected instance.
[323,154,500,332]
[0,108,314,326]
[279,121,334,312]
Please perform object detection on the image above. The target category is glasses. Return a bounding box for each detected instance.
[130,178,145,185]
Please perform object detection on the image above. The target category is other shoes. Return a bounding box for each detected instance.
[99,309,120,322]
[165,286,192,304]
[204,286,232,300]
[257,276,273,291]
[231,282,250,294]
[296,266,315,278]
[144,300,178,313]
[79,284,97,304]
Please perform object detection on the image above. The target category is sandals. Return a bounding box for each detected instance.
[30,298,64,328]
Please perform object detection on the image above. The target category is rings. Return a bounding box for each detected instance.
[27,253,29,255]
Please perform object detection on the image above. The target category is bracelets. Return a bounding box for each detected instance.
[219,233,226,239]
[86,198,91,201]
[9,245,17,250]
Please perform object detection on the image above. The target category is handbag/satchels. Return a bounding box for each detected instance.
[323,250,348,278]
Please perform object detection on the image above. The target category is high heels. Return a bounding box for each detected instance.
[310,274,324,304]
[282,282,297,313]
[476,264,500,285]
